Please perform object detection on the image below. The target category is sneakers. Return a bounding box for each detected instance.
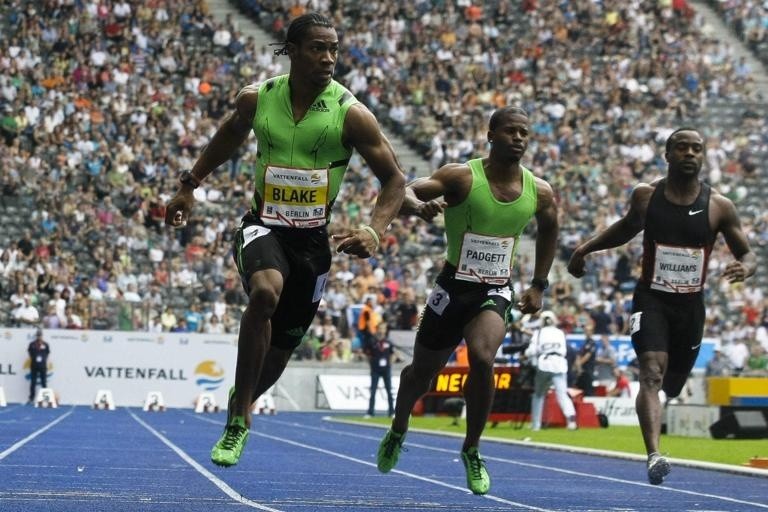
[460,446,490,495]
[377,423,408,473]
[212,386,251,468]
[647,452,671,484]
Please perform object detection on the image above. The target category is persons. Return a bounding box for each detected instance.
[364,320,399,420]
[521,310,578,430]
[153,10,412,464]
[373,102,559,500]
[23,325,50,402]
[565,123,757,487]
[0,0,768,396]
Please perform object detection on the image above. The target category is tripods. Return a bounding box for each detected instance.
[490,354,531,429]
